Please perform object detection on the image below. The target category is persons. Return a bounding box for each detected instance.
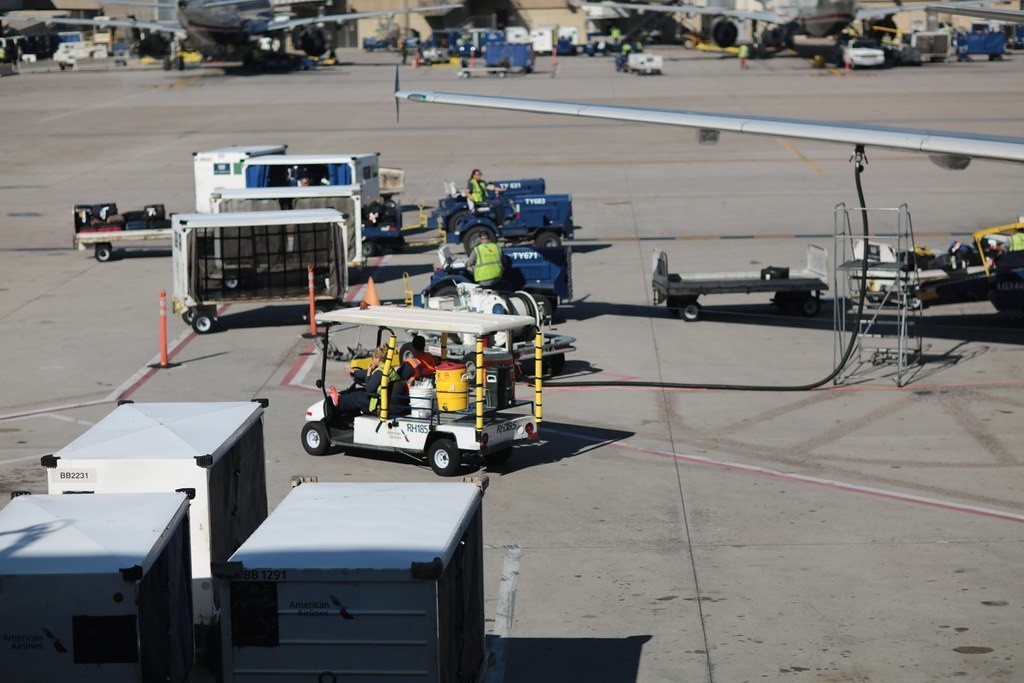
[468,230,504,287]
[466,169,507,202]
[392,336,436,395]
[337,347,401,414]
[1004,227,1024,282]
[294,178,310,186]
[738,45,750,69]
[611,24,621,34]
[620,41,632,57]
[636,38,643,52]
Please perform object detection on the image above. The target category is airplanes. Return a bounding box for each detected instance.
[565,0,1024,64]
[0,0,465,76]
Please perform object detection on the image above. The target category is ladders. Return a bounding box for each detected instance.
[832,201,925,389]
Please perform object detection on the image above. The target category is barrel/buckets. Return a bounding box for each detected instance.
[436,362,468,412]
[409,389,437,418]
[483,354,515,399]
[485,363,513,408]
